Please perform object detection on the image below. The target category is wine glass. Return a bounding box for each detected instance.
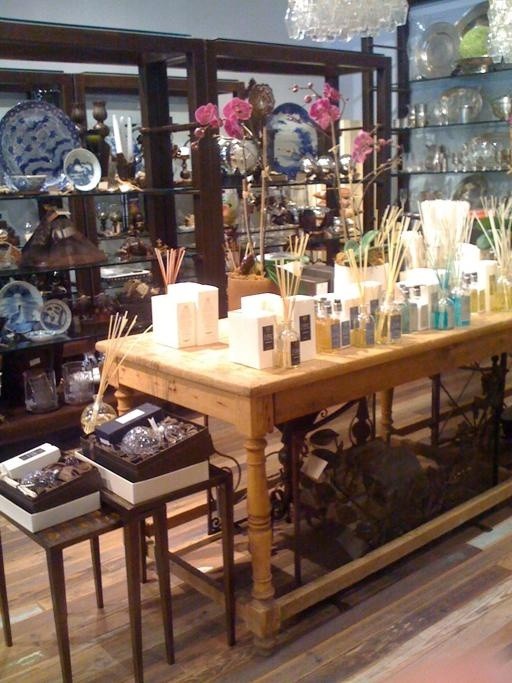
[446,139,507,172]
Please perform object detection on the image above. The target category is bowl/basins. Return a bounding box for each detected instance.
[454,53,493,74]
[9,174,48,193]
[62,147,103,190]
[22,330,55,342]
[490,94,512,120]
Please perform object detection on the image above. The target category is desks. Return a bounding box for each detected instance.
[95,311,512,656]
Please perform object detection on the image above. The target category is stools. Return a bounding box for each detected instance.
[88,462,240,663]
[2,501,142,682]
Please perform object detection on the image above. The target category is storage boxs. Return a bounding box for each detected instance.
[75,410,214,506]
[0,454,102,532]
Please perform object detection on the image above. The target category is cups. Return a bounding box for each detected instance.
[18,361,95,414]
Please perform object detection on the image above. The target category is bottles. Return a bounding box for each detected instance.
[278,272,489,368]
[404,103,428,127]
[21,455,81,492]
[121,420,186,458]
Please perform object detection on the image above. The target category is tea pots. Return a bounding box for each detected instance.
[423,143,445,172]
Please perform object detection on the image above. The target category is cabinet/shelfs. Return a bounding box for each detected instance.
[0,0,512,446]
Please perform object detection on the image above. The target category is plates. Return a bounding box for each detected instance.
[439,87,482,128]
[263,104,322,181]
[0,280,43,333]
[0,101,82,187]
[40,298,73,335]
[411,21,459,78]
